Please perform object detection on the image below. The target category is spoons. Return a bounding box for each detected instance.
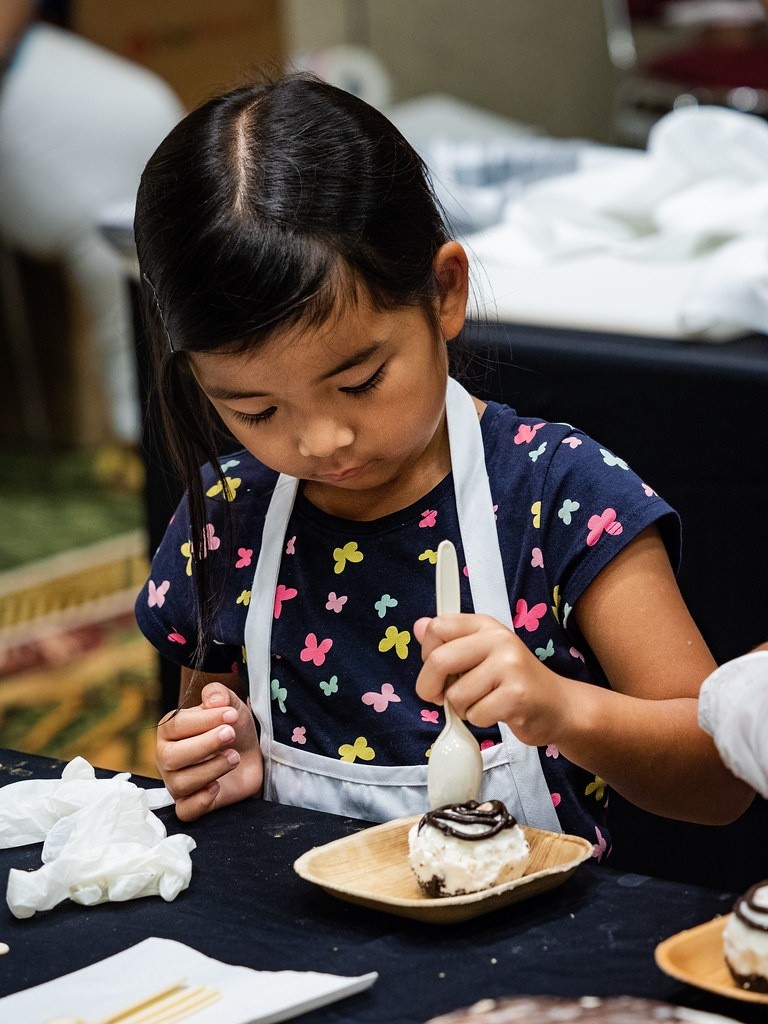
[425,540,484,811]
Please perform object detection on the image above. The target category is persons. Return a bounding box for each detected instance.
[131,76,754,869]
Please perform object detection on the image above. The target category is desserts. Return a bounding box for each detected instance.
[407,800,530,898]
[722,878,768,994]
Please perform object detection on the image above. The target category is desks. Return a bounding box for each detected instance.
[0,747,768,1024]
[121,266,768,721]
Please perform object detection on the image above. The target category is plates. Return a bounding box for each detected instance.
[654,913,768,1004]
[293,813,596,924]
[0,937,378,1024]
